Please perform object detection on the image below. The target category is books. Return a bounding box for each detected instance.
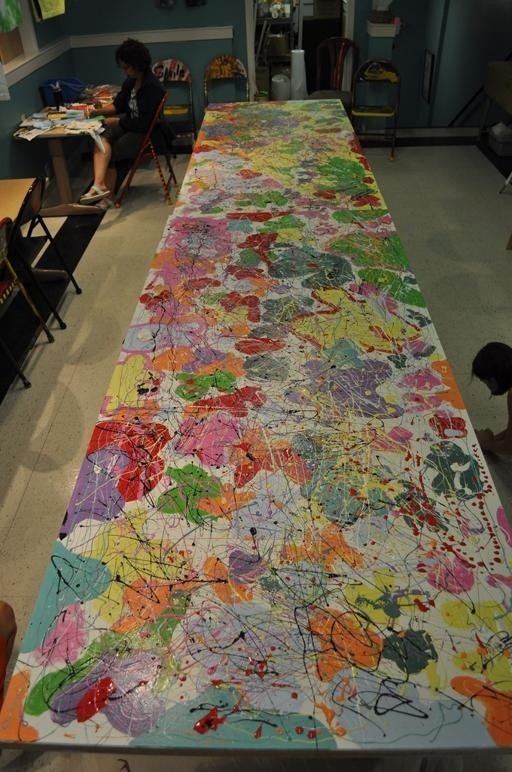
[14,82,121,142]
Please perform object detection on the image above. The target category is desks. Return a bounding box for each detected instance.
[1,99,510,750]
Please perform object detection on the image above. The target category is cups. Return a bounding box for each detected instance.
[53,88,64,111]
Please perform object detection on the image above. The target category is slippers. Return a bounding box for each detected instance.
[80,185,111,205]
[96,198,116,209]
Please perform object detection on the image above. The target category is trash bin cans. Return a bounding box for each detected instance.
[271,74,290,101]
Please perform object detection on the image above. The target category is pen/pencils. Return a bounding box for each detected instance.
[86,105,90,118]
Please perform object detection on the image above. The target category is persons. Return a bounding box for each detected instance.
[0,599,17,714]
[76,35,165,212]
[470,340,511,492]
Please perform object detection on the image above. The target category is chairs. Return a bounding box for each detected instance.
[309,37,400,160]
[0,61,250,388]
